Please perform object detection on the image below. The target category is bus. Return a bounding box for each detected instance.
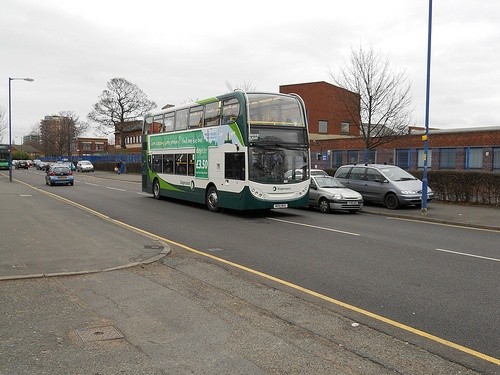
[140,88,312,213]
[0,145,10,170]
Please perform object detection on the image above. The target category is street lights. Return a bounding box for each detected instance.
[8,77,34,181]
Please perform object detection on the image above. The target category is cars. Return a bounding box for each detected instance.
[12,159,32,170]
[33,159,76,186]
[284,169,364,213]
[77,161,95,173]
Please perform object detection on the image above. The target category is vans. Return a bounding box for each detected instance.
[334,163,434,209]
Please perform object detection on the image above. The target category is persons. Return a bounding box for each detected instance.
[116,160,126,174]
